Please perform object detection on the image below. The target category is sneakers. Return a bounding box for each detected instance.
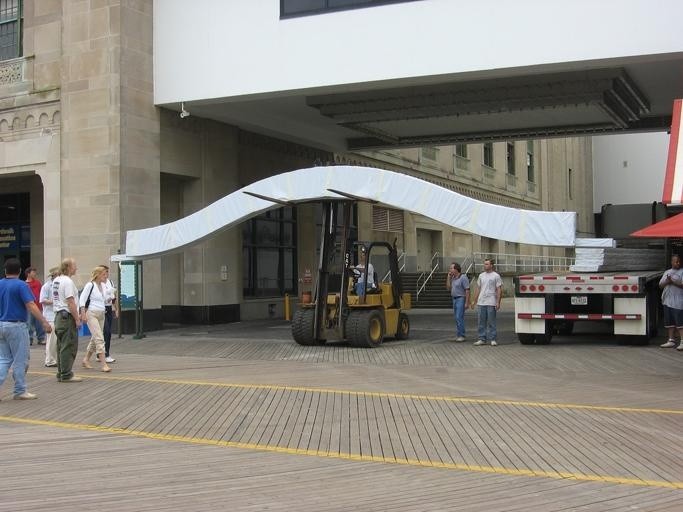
[491,340,496,346]
[96,356,114,371]
[456,336,464,341]
[474,340,486,345]
[661,341,683,350]
[81,360,92,369]
[13,392,37,400]
[57,376,81,382]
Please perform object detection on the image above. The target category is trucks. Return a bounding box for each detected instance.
[512,202,683,351]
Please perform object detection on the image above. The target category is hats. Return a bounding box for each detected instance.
[48,266,59,276]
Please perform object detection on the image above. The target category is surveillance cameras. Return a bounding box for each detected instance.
[180,112,190,118]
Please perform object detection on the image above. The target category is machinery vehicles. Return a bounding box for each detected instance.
[239,188,413,349]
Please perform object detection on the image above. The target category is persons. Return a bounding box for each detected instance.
[470,258,504,346]
[446,262,470,342]
[350,249,375,296]
[0,256,120,400]
[659,254,683,350]
